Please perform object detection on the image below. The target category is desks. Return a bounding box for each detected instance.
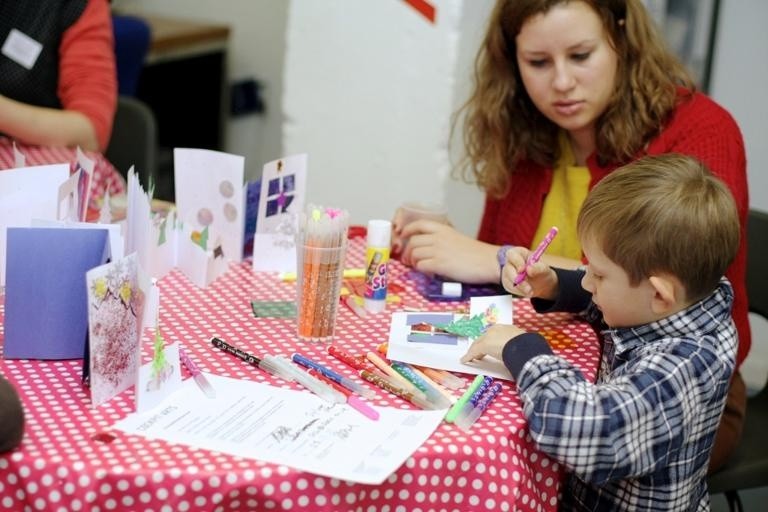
[1,141,131,223]
[3,231,600,510]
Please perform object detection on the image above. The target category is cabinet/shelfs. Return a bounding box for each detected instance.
[113,47,224,203]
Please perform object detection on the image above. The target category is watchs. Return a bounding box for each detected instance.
[495,242,518,292]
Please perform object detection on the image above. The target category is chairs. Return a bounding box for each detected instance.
[706,207,768,512]
[102,95,156,197]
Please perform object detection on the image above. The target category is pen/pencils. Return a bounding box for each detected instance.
[212,338,504,432]
[512,225,559,289]
[176,348,217,403]
[295,204,345,342]
[342,294,369,320]
[277,268,366,282]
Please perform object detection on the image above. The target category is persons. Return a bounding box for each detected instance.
[391,0,751,480]
[459,149,742,509]
[0,0,121,155]
